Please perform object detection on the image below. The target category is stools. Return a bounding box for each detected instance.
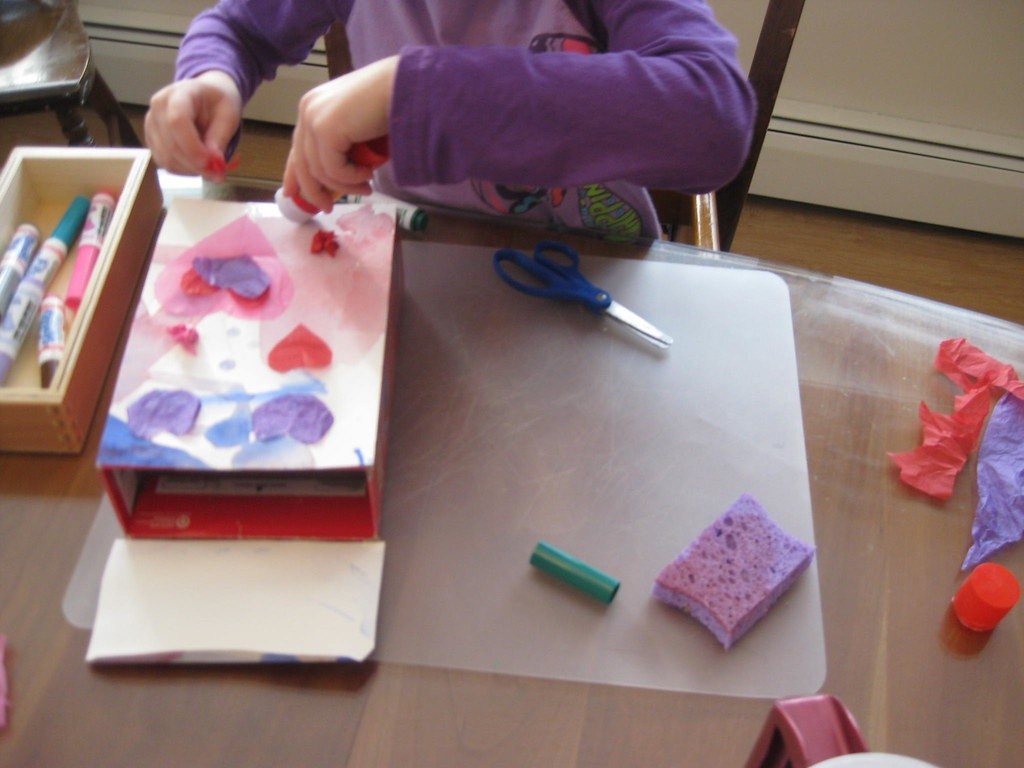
[0,0,143,149]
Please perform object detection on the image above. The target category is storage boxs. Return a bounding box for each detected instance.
[0,146,163,455]
[85,198,398,669]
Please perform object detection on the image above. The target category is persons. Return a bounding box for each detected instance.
[144,1,756,239]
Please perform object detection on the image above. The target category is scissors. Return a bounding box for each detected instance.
[493,241,676,349]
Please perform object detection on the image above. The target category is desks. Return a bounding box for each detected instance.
[0,178,1024,768]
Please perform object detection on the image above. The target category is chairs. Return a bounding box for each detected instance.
[325,0,806,250]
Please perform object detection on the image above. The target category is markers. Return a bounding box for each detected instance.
[0,184,116,385]
[340,186,428,233]
